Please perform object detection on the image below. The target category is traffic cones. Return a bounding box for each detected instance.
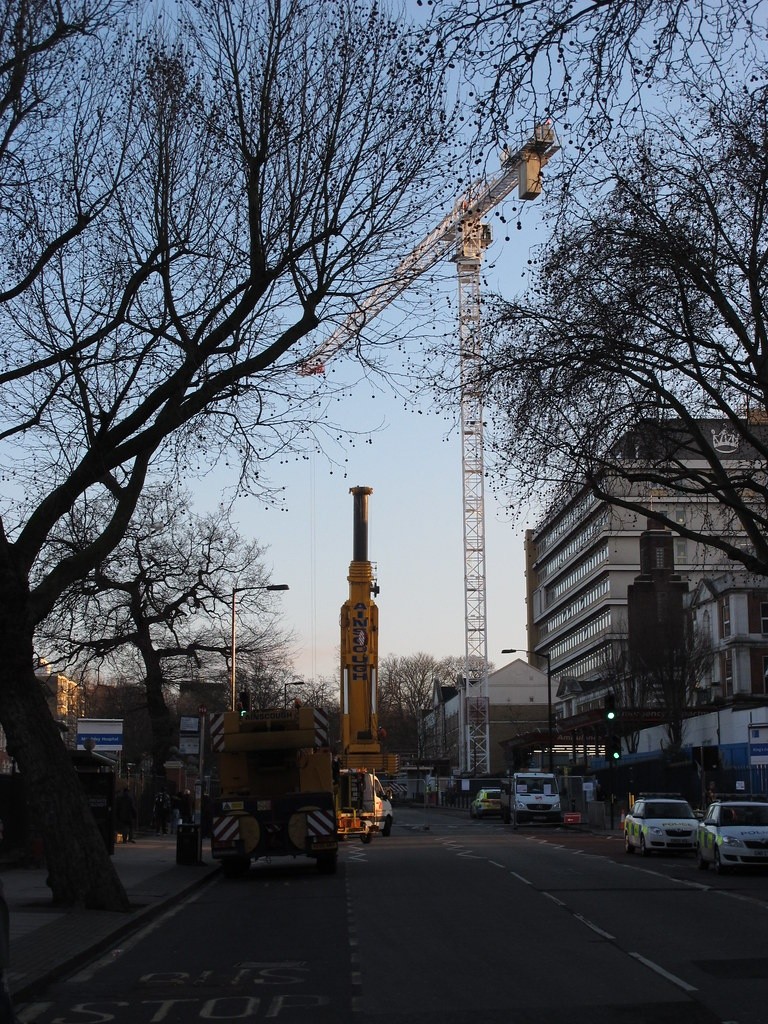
[620,809,627,831]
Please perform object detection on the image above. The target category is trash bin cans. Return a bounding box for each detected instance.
[175,822,202,866]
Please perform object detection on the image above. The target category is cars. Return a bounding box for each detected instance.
[470,787,503,819]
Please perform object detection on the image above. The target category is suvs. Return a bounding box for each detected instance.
[697,799,768,875]
[624,797,704,858]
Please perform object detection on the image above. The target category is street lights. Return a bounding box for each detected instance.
[501,650,555,754]
[231,585,290,712]
[284,681,305,709]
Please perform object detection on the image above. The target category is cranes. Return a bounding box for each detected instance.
[292,120,563,778]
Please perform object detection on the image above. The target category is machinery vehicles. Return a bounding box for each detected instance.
[323,485,408,805]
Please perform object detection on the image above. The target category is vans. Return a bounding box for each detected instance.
[502,772,563,823]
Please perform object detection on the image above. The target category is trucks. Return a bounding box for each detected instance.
[211,706,343,877]
[335,768,393,844]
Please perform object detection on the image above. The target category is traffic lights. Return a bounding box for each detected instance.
[239,692,251,711]
[605,695,617,726]
[610,735,621,761]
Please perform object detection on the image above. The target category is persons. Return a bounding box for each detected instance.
[152,784,195,837]
[705,781,715,808]
[120,788,138,844]
